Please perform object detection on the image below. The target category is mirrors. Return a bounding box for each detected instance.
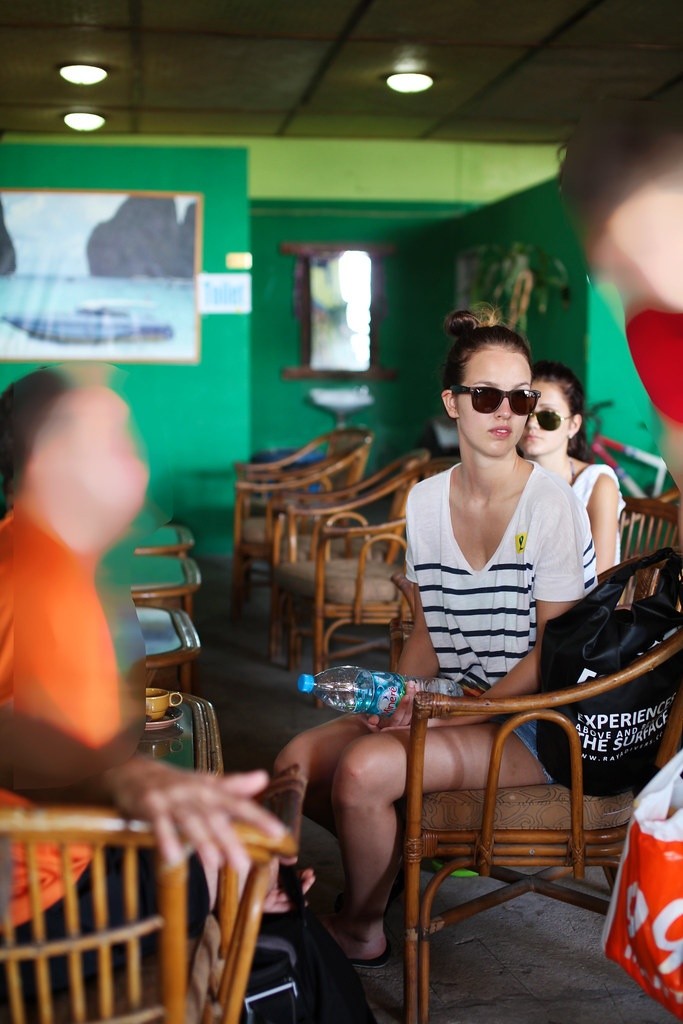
[278,239,399,382]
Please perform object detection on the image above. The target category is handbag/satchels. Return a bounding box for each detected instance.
[535,547,683,799]
[598,747,683,1021]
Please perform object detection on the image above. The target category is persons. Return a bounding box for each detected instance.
[518,360,626,576]
[270,302,598,967]
[0,370,375,1024]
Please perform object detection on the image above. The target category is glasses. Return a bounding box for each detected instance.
[449,385,541,416]
[526,411,561,430]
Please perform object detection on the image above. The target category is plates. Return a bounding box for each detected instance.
[145,707,184,732]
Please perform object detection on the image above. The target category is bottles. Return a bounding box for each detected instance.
[297,666,464,719]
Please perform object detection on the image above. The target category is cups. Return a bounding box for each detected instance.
[145,688,183,722]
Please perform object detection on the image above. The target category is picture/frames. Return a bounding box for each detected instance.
[0,186,201,366]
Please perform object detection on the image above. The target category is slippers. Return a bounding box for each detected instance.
[341,939,392,969]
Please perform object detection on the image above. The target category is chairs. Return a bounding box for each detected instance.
[1,804,298,1024]
[231,428,683,1024]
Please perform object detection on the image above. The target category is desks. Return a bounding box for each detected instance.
[122,525,225,779]
